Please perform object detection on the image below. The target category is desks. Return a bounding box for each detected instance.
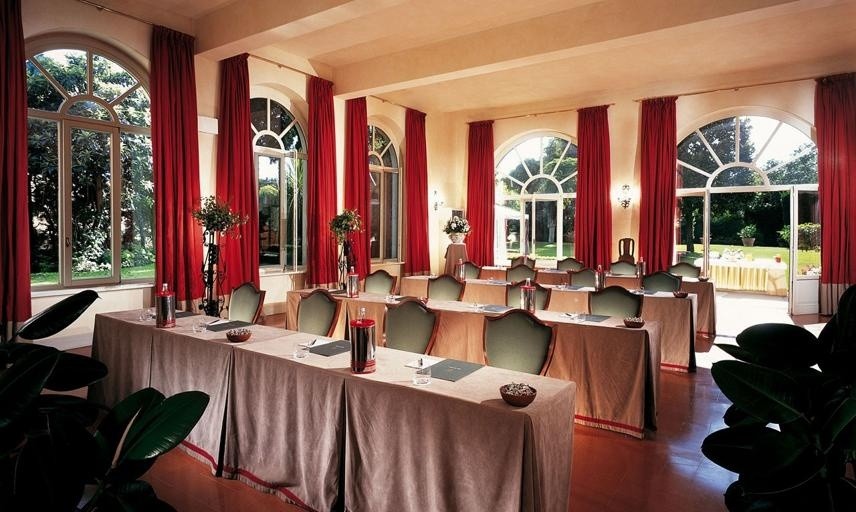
[286,286,663,440]
[478,265,716,351]
[399,273,699,375]
[88,306,577,512]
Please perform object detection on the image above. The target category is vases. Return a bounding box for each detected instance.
[449,234,466,245]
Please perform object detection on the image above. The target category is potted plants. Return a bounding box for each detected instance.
[328,206,369,258]
[738,224,758,247]
[193,193,250,265]
[694,254,787,292]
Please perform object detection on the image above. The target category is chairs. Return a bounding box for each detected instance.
[504,282,554,307]
[669,261,701,276]
[227,280,268,327]
[381,298,439,356]
[568,267,602,286]
[294,289,346,337]
[506,266,540,280]
[426,272,468,299]
[455,261,481,278]
[587,284,646,316]
[611,260,638,275]
[482,309,555,376]
[556,256,584,272]
[511,255,537,267]
[640,269,685,291]
[366,267,397,293]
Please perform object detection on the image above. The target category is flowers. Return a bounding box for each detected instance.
[443,215,472,234]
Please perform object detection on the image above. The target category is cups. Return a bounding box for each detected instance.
[192,318,207,334]
[412,368,432,385]
[136,309,147,322]
[575,312,586,323]
[297,345,311,358]
[145,309,153,321]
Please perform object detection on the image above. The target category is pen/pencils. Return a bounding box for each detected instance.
[312,339,317,345]
[565,312,572,317]
[474,302,477,308]
[210,319,220,324]
[421,358,423,367]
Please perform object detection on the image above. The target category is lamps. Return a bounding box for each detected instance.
[433,189,446,211]
[617,183,631,210]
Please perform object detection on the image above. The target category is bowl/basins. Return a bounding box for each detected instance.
[698,277,709,282]
[674,291,688,298]
[500,383,537,408]
[226,333,251,343]
[623,319,645,328]
